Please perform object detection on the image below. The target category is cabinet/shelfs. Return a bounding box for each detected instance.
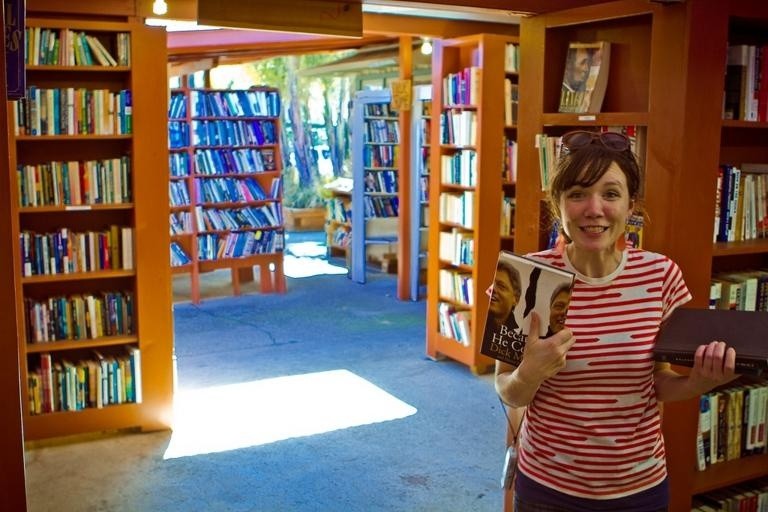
[325,0,764,512]
[7,19,182,443]
[167,84,287,307]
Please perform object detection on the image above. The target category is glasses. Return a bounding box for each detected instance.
[559,128,633,160]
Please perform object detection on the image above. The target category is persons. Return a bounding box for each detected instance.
[494,133,744,511]
[539,283,573,338]
[481,262,526,366]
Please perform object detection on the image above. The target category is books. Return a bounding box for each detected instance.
[419,66,478,344]
[328,102,400,248]
[499,42,643,255]
[12,23,142,424]
[697,45,768,510]
[655,308,766,385]
[477,249,576,372]
[166,89,281,266]
